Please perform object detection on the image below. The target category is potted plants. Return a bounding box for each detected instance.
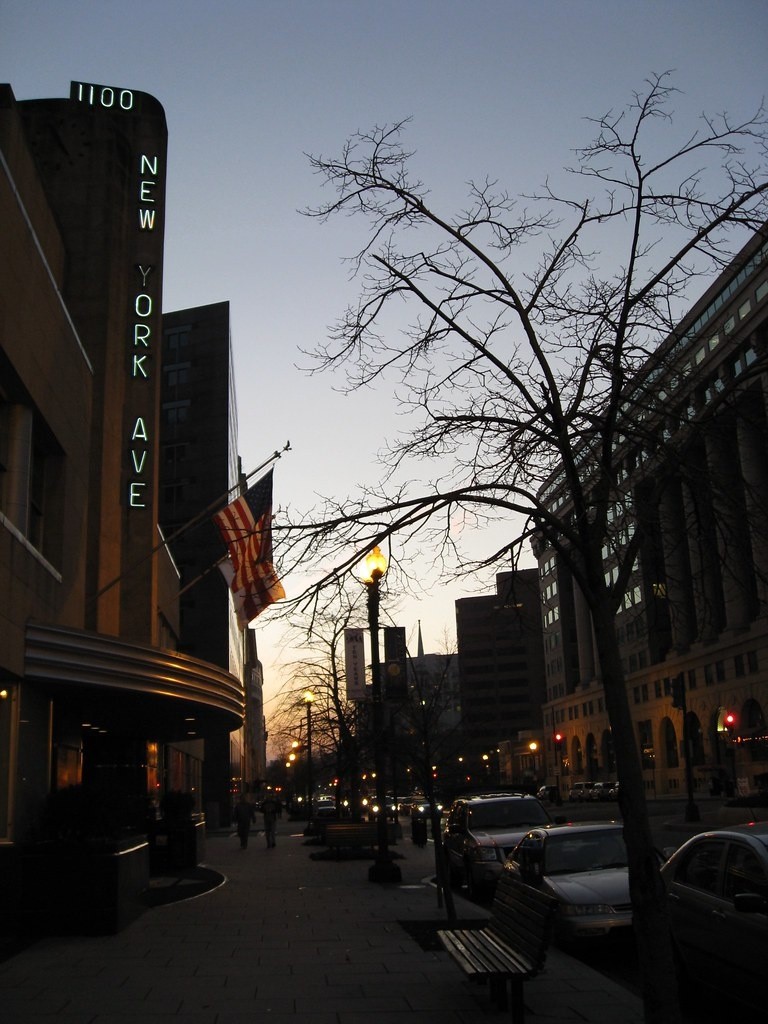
[151,789,207,870]
[36,780,153,936]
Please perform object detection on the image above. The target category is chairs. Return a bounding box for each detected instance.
[598,835,622,864]
[554,843,578,871]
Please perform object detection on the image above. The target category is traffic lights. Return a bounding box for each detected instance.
[669,677,679,709]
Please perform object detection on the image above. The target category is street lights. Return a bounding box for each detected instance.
[555,733,563,741]
[529,742,538,775]
[736,735,742,745]
[725,714,735,725]
[363,543,403,887]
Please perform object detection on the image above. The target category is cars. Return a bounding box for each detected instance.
[316,794,444,819]
[658,819,768,1003]
[502,817,671,954]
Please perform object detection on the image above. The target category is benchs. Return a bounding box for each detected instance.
[436,873,561,1024]
[311,817,399,862]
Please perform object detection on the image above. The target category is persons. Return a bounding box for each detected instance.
[231,793,256,849]
[259,796,277,848]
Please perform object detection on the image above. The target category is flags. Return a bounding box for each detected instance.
[211,467,285,631]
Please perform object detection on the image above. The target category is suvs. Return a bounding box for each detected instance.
[536,784,558,801]
[568,780,594,803]
[444,792,557,905]
[588,781,616,803]
[608,781,620,800]
